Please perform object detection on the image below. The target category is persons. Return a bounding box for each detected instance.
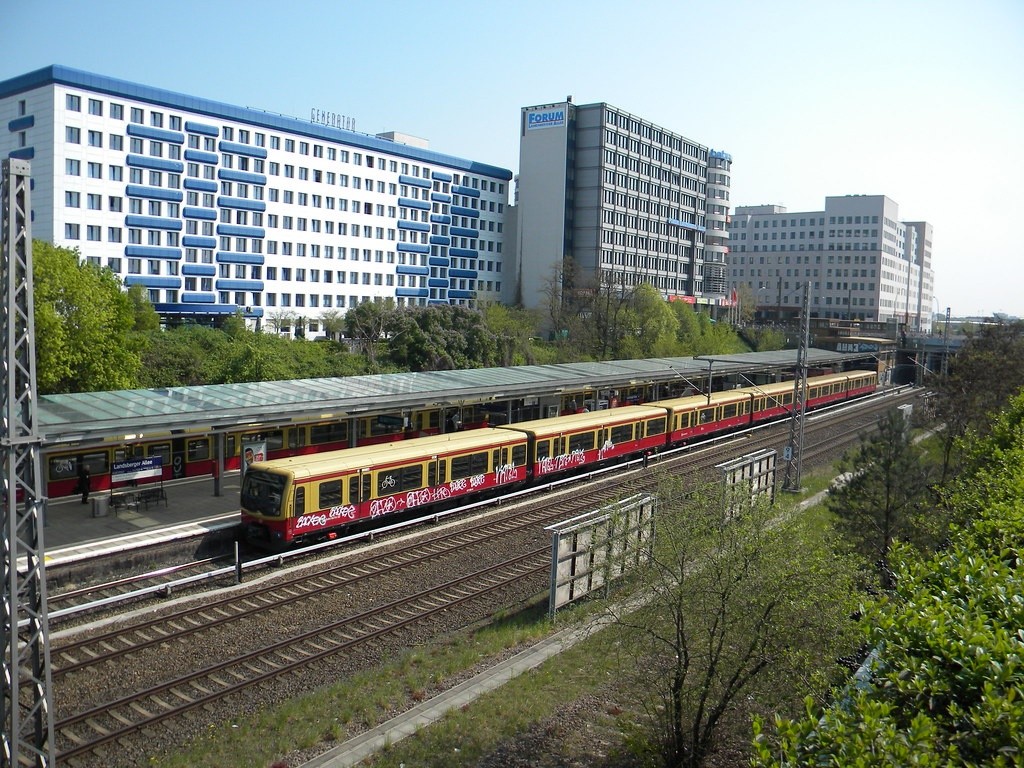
[79,464,91,504]
[452,412,462,431]
[572,399,578,413]
[701,412,706,421]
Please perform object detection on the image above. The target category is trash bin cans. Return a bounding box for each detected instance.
[92,495,109,517]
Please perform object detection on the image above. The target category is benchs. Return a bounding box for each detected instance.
[111,488,168,516]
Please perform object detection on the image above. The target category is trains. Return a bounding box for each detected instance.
[0,346,845,509]
[241,371,880,555]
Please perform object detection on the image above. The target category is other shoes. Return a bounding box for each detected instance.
[82,501,89,504]
